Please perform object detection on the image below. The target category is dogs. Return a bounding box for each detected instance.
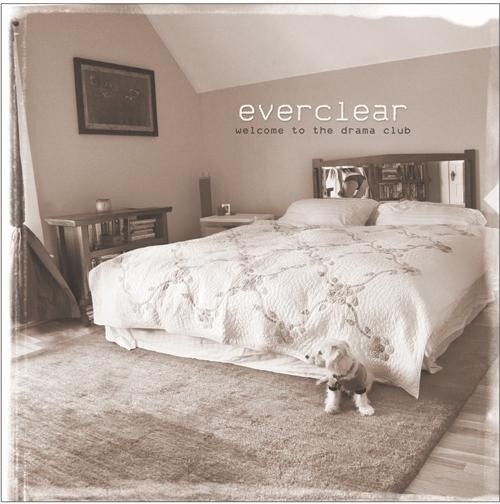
[304,339,376,418]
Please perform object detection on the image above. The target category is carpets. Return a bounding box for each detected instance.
[11,333,499,502]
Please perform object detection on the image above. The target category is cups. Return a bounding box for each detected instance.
[95,199,111,212]
[217,207,225,216]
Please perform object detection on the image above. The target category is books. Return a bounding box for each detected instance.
[377,162,426,199]
[82,217,156,324]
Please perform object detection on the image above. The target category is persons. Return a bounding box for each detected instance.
[335,167,367,198]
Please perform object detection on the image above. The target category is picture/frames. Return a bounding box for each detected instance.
[221,204,230,216]
[74,56,158,136]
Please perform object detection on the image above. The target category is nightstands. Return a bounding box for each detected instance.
[201,213,275,240]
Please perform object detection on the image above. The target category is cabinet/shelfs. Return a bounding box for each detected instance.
[45,206,173,329]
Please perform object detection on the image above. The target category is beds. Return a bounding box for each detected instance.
[88,150,499,400]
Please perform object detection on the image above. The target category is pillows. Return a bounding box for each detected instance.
[279,197,379,229]
[376,202,487,228]
